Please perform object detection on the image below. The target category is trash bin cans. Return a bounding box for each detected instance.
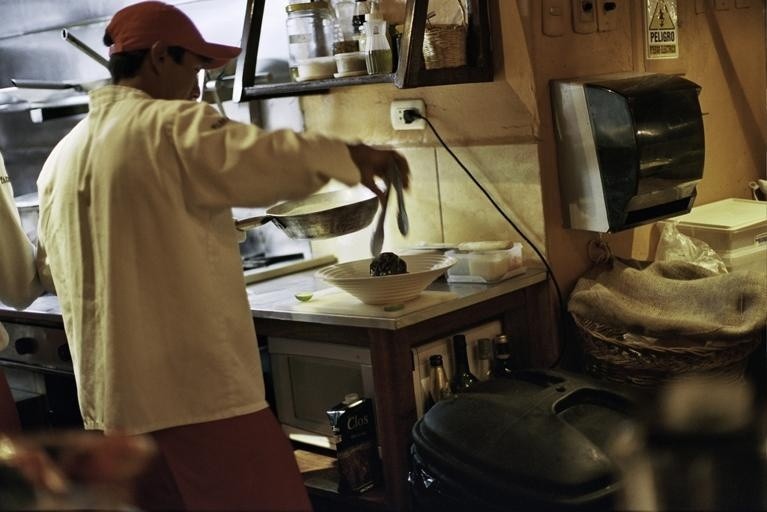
[410,369,650,512]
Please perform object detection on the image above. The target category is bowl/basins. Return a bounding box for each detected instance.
[315,254,450,309]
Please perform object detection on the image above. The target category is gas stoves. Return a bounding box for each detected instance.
[0,251,334,377]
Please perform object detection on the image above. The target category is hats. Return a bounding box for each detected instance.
[107,1,242,68]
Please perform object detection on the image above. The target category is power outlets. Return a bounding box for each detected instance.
[391,99,426,130]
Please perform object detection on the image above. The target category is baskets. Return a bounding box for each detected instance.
[571,255,766,392]
[422,0,468,68]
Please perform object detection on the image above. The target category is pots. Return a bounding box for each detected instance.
[7,75,112,96]
[234,187,380,238]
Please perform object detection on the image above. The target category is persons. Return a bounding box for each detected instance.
[36,1,409,512]
[0,148,46,443]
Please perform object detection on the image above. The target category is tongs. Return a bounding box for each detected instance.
[367,173,406,254]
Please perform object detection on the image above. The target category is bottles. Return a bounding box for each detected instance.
[420,332,522,401]
[279,3,397,81]
